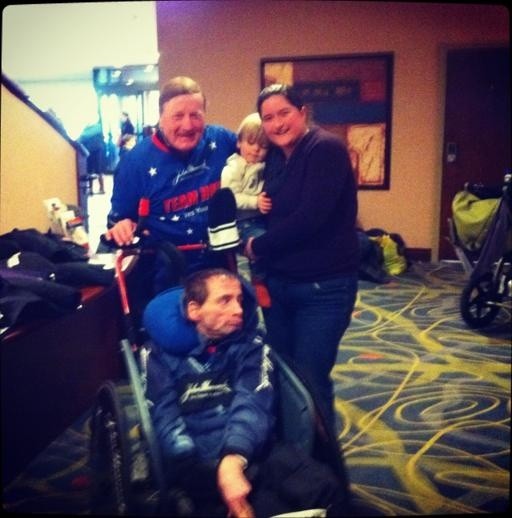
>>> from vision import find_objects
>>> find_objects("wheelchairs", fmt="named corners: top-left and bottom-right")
top-left (94, 230), bottom-right (349, 515)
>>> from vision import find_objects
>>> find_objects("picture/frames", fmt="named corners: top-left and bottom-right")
top-left (259, 51), bottom-right (394, 191)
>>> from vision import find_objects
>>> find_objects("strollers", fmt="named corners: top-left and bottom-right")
top-left (443, 172), bottom-right (512, 329)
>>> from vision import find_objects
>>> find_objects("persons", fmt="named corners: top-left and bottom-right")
top-left (219, 114), bottom-right (273, 309)
top-left (120, 112), bottom-right (135, 136)
top-left (147, 266), bottom-right (350, 518)
top-left (105, 76), bottom-right (240, 279)
top-left (241, 84), bottom-right (359, 434)
